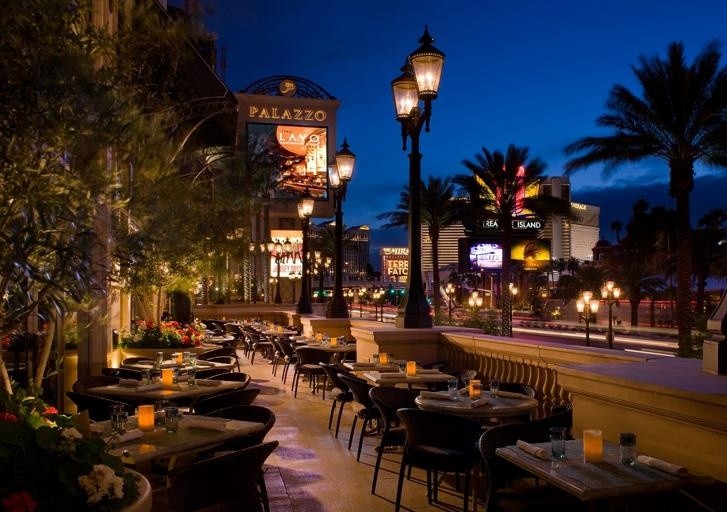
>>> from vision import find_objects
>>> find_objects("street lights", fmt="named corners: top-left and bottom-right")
top-left (445, 282), bottom-right (456, 325)
top-left (342, 287), bottom-right (387, 323)
top-left (575, 289), bottom-right (600, 348)
top-left (468, 290), bottom-right (483, 308)
top-left (325, 136), bottom-right (357, 318)
top-left (599, 280), bottom-right (622, 350)
top-left (390, 21), bottom-right (447, 329)
top-left (296, 185), bottom-right (316, 315)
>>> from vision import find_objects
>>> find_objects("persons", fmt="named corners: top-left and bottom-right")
top-left (275, 239), bottom-right (283, 264)
top-left (283, 238), bottom-right (293, 264)
top-left (292, 239), bottom-right (302, 265)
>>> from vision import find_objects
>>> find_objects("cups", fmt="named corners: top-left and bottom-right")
top-left (166, 407), bottom-right (178, 434)
top-left (151, 369), bottom-right (161, 383)
top-left (157, 352), bottom-right (164, 364)
top-left (398, 360), bottom-right (407, 375)
top-left (171, 354), bottom-right (178, 367)
top-left (141, 372), bottom-right (150, 385)
top-left (386, 352), bottom-right (394, 364)
top-left (115, 412), bottom-right (128, 434)
top-left (448, 377), bottom-right (457, 400)
top-left (373, 352), bottom-right (380, 367)
top-left (473, 384), bottom-right (483, 400)
top-left (172, 370), bottom-right (179, 383)
top-left (488, 380), bottom-right (499, 400)
top-left (550, 427), bottom-right (566, 458)
top-left (183, 351), bottom-right (190, 363)
top-left (187, 370), bottom-right (196, 384)
top-left (617, 433), bottom-right (637, 464)
top-left (190, 353), bottom-right (196, 367)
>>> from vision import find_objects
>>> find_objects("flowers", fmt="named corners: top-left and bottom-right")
top-left (0, 385), bottom-right (140, 512)
